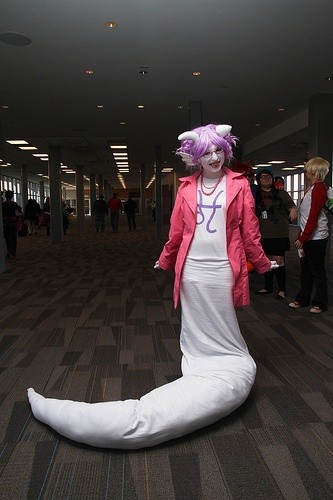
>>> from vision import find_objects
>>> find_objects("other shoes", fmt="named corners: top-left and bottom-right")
top-left (309, 306), bottom-right (322, 313)
top-left (254, 289), bottom-right (273, 295)
top-left (288, 301), bottom-right (301, 307)
top-left (275, 291), bottom-right (286, 300)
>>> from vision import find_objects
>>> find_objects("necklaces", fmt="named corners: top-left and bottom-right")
top-left (201, 172), bottom-right (225, 196)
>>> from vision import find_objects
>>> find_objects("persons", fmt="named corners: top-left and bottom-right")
top-left (152, 201), bottom-right (156, 222)
top-left (232, 156), bottom-right (333, 313)
top-left (124, 194), bottom-right (137, 231)
top-left (92, 195), bottom-right (109, 232)
top-left (26, 123), bottom-right (280, 449)
top-left (0, 190), bottom-right (72, 259)
top-left (107, 193), bottom-right (123, 232)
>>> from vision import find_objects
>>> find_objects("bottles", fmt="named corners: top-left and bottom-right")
top-left (295, 240), bottom-right (306, 263)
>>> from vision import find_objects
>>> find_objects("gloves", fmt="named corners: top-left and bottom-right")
top-left (153, 260), bottom-right (161, 270)
top-left (266, 260), bottom-right (279, 272)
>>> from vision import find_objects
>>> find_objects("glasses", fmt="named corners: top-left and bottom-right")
top-left (261, 175), bottom-right (271, 179)
top-left (197, 148), bottom-right (223, 159)
